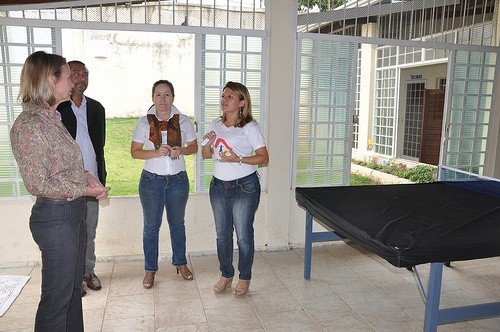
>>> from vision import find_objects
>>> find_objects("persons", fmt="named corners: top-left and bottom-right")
top-left (201, 82), bottom-right (269, 298)
top-left (9, 51), bottom-right (111, 332)
top-left (55, 61), bottom-right (105, 295)
top-left (131, 81), bottom-right (199, 289)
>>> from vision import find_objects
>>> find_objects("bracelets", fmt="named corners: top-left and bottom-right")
top-left (239, 155), bottom-right (242, 165)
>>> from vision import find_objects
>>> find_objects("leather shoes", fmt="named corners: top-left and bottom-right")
top-left (83, 273), bottom-right (101, 290)
top-left (82, 289), bottom-right (86, 297)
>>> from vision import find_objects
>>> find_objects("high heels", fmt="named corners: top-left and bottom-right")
top-left (213, 277), bottom-right (232, 292)
top-left (235, 280), bottom-right (250, 297)
top-left (143, 272), bottom-right (155, 288)
top-left (176, 265), bottom-right (193, 280)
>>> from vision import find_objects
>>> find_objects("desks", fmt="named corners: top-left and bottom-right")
top-left (294, 179), bottom-right (500, 332)
